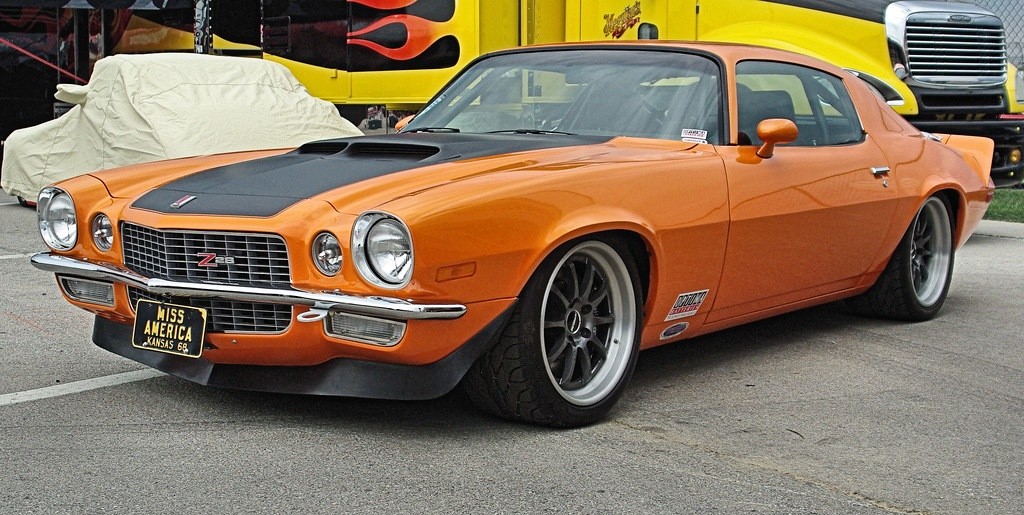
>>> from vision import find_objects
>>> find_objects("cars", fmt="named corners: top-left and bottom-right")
top-left (2, 52), bottom-right (363, 210)
top-left (29, 37), bottom-right (995, 431)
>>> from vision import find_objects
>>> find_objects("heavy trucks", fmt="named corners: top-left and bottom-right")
top-left (0, 0), bottom-right (1022, 194)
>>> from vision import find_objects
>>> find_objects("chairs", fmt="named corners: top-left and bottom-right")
top-left (560, 77), bottom-right (800, 144)
